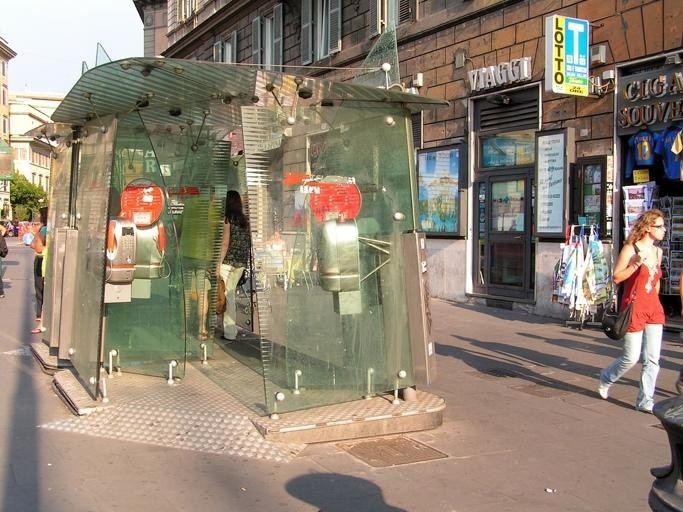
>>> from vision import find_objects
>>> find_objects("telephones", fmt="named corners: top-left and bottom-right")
top-left (106, 220), bottom-right (136, 268)
top-left (158, 220), bottom-right (165, 252)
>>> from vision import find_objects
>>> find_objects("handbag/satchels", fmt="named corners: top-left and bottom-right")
top-left (600, 305), bottom-right (634, 338)
top-left (210, 276), bottom-right (226, 315)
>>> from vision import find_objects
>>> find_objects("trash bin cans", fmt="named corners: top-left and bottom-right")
top-left (18, 221), bottom-right (42, 243)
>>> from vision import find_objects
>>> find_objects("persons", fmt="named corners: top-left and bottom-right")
top-left (261, 230), bottom-right (287, 256)
top-left (175, 185), bottom-right (219, 342)
top-left (29, 232), bottom-right (46, 334)
top-left (0, 224), bottom-right (7, 298)
top-left (598, 209), bottom-right (666, 414)
top-left (674, 268), bottom-right (682, 397)
top-left (214, 190), bottom-right (251, 341)
top-left (26, 207), bottom-right (47, 321)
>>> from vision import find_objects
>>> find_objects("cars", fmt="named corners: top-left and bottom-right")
top-left (0, 219), bottom-right (44, 240)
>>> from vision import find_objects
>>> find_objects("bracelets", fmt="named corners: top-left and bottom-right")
top-left (215, 263), bottom-right (220, 268)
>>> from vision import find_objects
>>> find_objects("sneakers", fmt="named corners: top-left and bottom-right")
top-left (635, 398), bottom-right (655, 414)
top-left (182, 330), bottom-right (235, 341)
top-left (30, 327), bottom-right (45, 335)
top-left (597, 374), bottom-right (610, 399)
